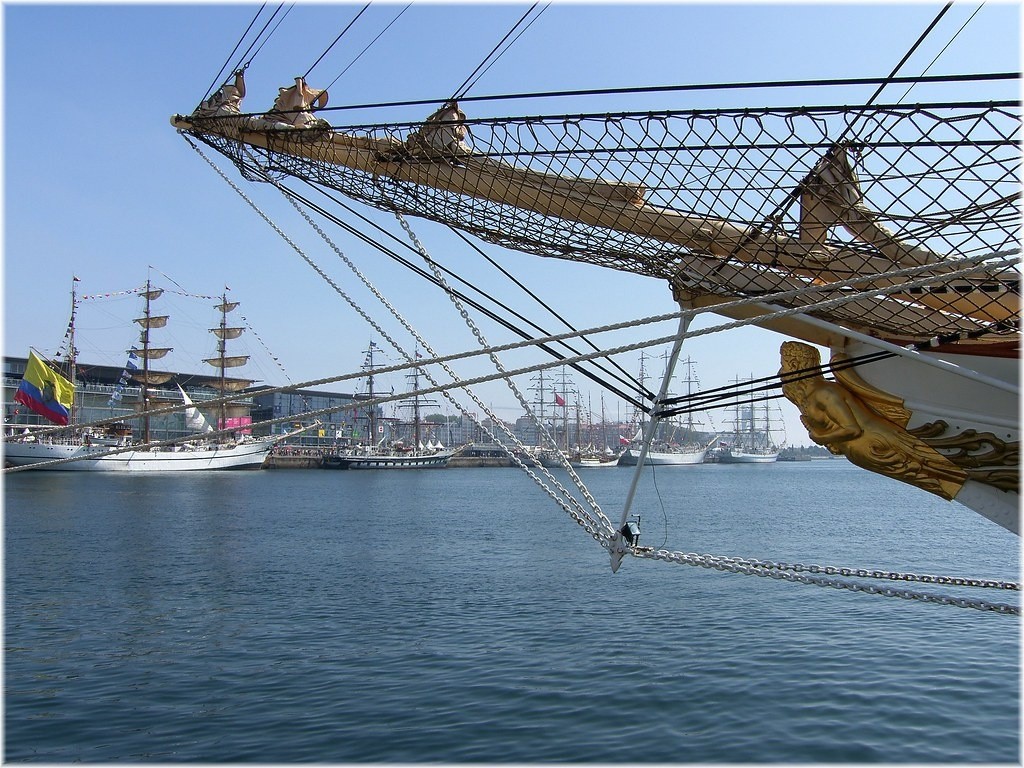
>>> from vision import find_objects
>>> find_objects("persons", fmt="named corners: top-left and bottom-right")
top-left (126, 438), bottom-right (145, 448)
top-left (482, 452), bottom-right (496, 457)
top-left (271, 446), bottom-right (333, 456)
top-left (87, 440), bottom-right (92, 447)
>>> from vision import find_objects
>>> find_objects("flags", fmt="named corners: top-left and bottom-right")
top-left (120, 378), bottom-right (127, 384)
top-left (619, 434), bottom-right (629, 445)
top-left (130, 352), bottom-right (138, 361)
top-left (116, 385), bottom-right (124, 392)
top-left (123, 370), bottom-right (132, 378)
top-left (111, 391), bottom-right (123, 401)
top-left (126, 361), bottom-right (137, 370)
top-left (105, 399), bottom-right (116, 406)
top-left (555, 392), bottom-right (565, 407)
top-left (13, 352), bottom-right (77, 426)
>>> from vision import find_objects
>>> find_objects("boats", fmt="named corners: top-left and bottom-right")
top-left (509, 347), bottom-right (787, 469)
top-left (262, 339), bottom-right (462, 469)
top-left (4, 264), bottom-right (320, 478)
